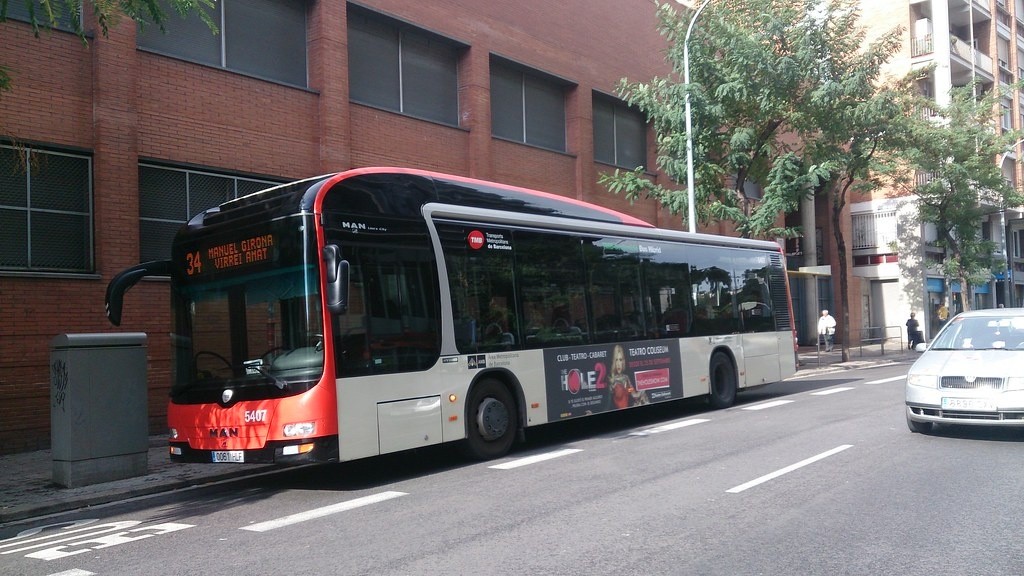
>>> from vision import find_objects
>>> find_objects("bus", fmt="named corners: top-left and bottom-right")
top-left (103, 164), bottom-right (802, 468)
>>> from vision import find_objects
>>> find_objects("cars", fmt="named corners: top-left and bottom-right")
top-left (906, 308), bottom-right (1024, 433)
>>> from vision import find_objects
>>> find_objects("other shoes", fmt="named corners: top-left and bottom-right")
top-left (908, 345), bottom-right (916, 350)
top-left (825, 349), bottom-right (834, 352)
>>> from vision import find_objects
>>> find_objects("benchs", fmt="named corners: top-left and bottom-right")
top-left (530, 317), bottom-right (582, 336)
top-left (479, 323), bottom-right (514, 345)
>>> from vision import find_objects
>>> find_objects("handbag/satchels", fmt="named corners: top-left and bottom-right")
top-left (826, 327), bottom-right (836, 335)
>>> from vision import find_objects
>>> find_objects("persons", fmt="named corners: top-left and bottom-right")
top-left (817, 310), bottom-right (837, 352)
top-left (936, 303), bottom-right (949, 330)
top-left (906, 313), bottom-right (919, 350)
top-left (609, 345), bottom-right (636, 409)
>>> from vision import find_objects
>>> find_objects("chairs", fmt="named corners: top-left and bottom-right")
top-left (366, 299), bottom-right (401, 345)
